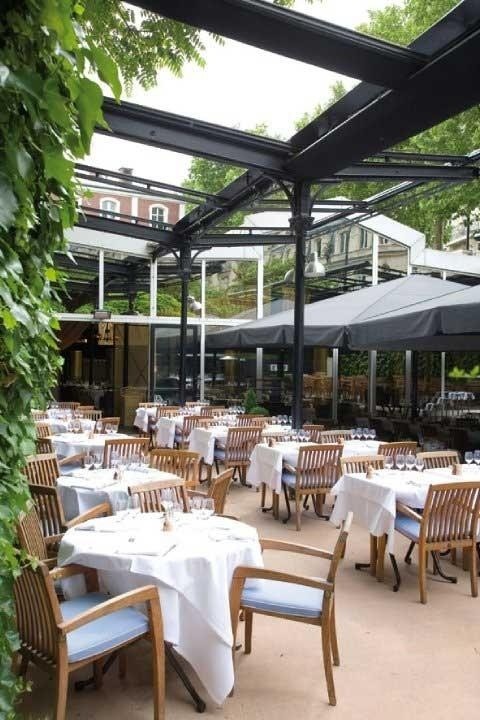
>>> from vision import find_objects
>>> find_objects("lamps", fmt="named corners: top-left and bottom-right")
top-left (187, 296), bottom-right (201, 310)
top-left (284, 264), bottom-right (296, 284)
top-left (303, 252), bottom-right (326, 278)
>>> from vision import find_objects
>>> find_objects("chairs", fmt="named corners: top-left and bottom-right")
top-left (63, 502), bottom-right (111, 531)
top-left (4, 545), bottom-right (167, 720)
top-left (11, 500), bottom-right (65, 570)
top-left (416, 450), bottom-right (460, 470)
top-left (377, 482), bottom-right (480, 603)
top-left (224, 512), bottom-right (354, 705)
top-left (26, 484), bottom-right (63, 539)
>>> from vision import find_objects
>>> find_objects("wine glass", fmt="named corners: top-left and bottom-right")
top-left (369, 429), bottom-right (376, 443)
top-left (416, 455), bottom-right (425, 476)
top-left (465, 451), bottom-right (474, 469)
top-left (357, 427), bottom-right (363, 443)
top-left (363, 427), bottom-right (369, 443)
top-left (407, 455), bottom-right (416, 473)
top-left (396, 455), bottom-right (406, 474)
top-left (474, 450), bottom-right (480, 469)
top-left (45, 398), bottom-right (312, 530)
top-left (384, 456), bottom-right (394, 474)
top-left (350, 428), bottom-right (357, 443)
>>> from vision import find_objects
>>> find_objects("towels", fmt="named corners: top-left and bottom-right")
top-left (115, 541), bottom-right (175, 557)
